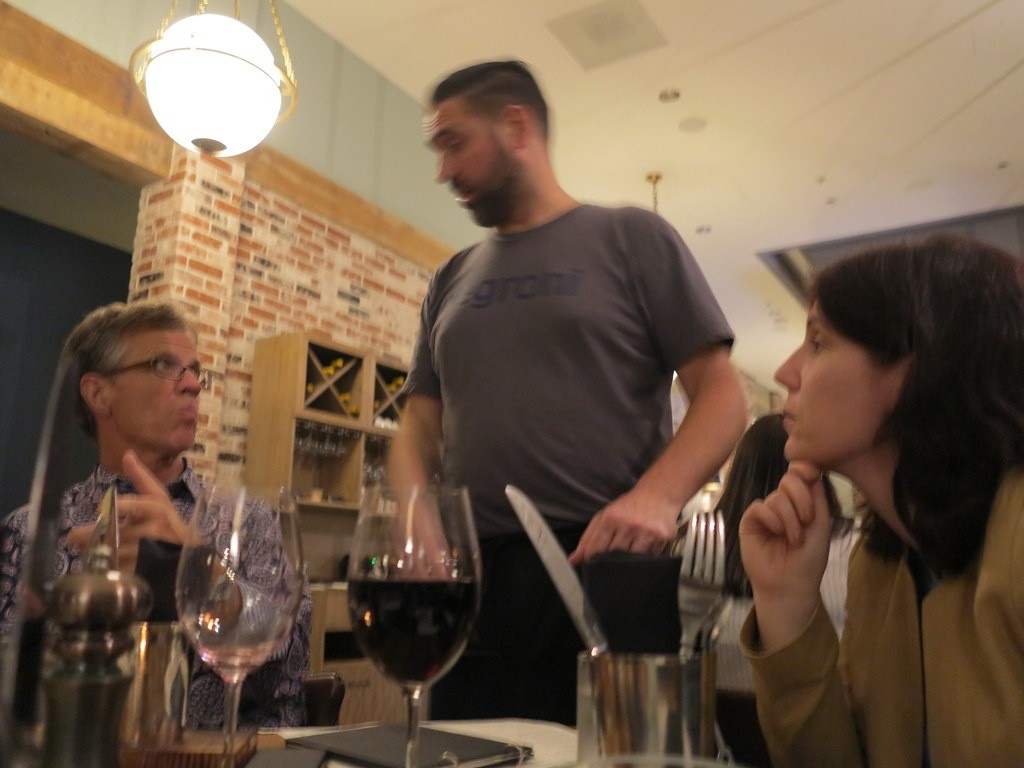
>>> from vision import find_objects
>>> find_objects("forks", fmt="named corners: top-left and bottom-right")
top-left (677, 509), bottom-right (725, 655)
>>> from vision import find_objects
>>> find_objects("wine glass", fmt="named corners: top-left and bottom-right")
top-left (174, 485), bottom-right (304, 768)
top-left (347, 485), bottom-right (482, 768)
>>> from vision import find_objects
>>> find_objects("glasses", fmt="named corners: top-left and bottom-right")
top-left (102, 353), bottom-right (213, 394)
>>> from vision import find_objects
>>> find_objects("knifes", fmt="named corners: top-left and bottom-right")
top-left (504, 484), bottom-right (609, 657)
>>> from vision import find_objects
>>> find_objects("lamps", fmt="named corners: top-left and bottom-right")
top-left (129, 0), bottom-right (299, 157)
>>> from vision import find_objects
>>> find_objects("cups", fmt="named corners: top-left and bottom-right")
top-left (576, 651), bottom-right (717, 768)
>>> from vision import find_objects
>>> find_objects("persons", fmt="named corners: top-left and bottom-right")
top-left (678, 234), bottom-right (1024, 768)
top-left (390, 59), bottom-right (748, 730)
top-left (0, 302), bottom-right (314, 730)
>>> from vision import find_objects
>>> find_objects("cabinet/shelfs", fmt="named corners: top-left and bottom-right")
top-left (243, 333), bottom-right (411, 677)
